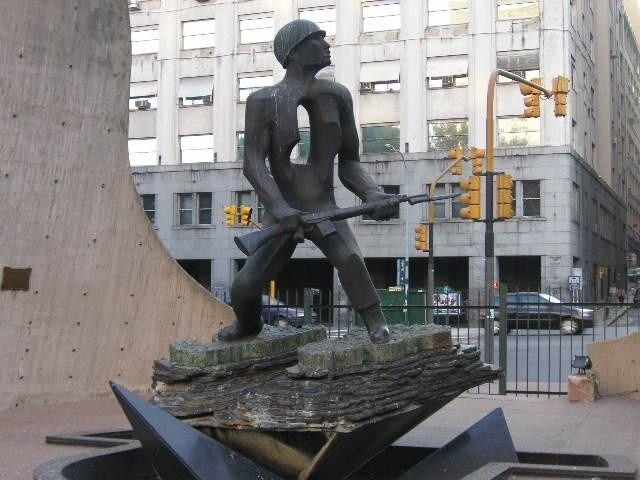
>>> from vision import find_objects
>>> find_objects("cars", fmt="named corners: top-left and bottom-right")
top-left (480, 290), bottom-right (595, 336)
top-left (225, 292), bottom-right (319, 332)
top-left (627, 267), bottom-right (640, 306)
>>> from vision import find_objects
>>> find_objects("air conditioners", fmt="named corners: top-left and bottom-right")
top-left (135, 99), bottom-right (149, 109)
top-left (361, 82), bottom-right (372, 90)
top-left (513, 70), bottom-right (526, 82)
top-left (442, 74), bottom-right (455, 87)
top-left (203, 94), bottom-right (214, 104)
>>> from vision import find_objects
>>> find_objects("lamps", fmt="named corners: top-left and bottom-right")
top-left (571, 353), bottom-right (593, 375)
top-left (384, 142), bottom-right (409, 169)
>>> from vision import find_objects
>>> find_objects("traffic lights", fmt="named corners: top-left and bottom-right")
top-left (413, 222), bottom-right (427, 250)
top-left (223, 205), bottom-right (236, 226)
top-left (240, 204), bottom-right (253, 226)
top-left (555, 75), bottom-right (571, 117)
top-left (496, 173), bottom-right (514, 219)
top-left (471, 145), bottom-right (486, 175)
top-left (520, 77), bottom-right (541, 118)
top-left (459, 173), bottom-right (480, 219)
top-left (447, 146), bottom-right (462, 177)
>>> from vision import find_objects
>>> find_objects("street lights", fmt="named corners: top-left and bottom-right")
top-left (382, 143), bottom-right (413, 323)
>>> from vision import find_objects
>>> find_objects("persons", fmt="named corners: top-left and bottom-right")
top-left (609, 284), bottom-right (618, 302)
top-left (618, 283), bottom-right (625, 302)
top-left (218, 21), bottom-right (400, 343)
